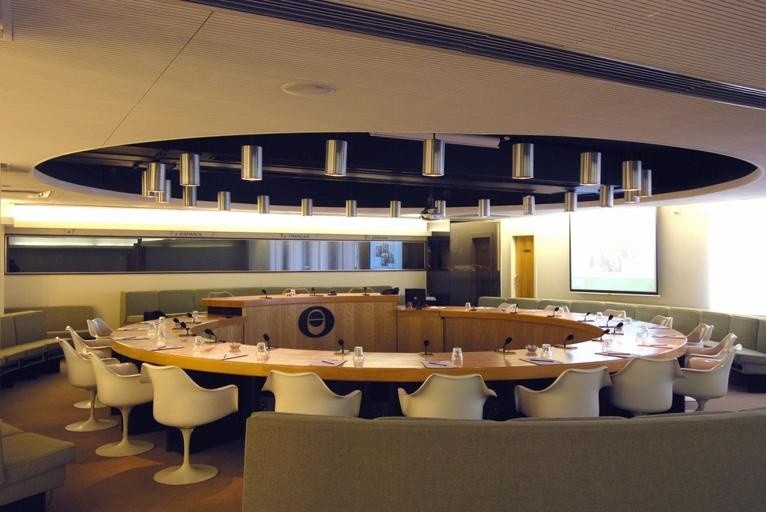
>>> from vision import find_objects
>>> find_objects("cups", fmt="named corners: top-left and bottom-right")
top-left (191, 336), bottom-right (204, 356)
top-left (255, 342), bottom-right (266, 355)
top-left (352, 345), bottom-right (363, 359)
top-left (407, 302), bottom-right (412, 309)
top-left (542, 343), bottom-right (552, 357)
top-left (595, 311), bottom-right (649, 352)
top-left (148, 316), bottom-right (167, 344)
top-left (557, 307), bottom-right (564, 316)
top-left (451, 346), bottom-right (464, 361)
top-left (510, 305), bottom-right (515, 312)
top-left (465, 302), bottom-right (471, 311)
top-left (191, 310), bottom-right (199, 318)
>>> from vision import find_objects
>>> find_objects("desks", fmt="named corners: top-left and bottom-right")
top-left (111, 302), bottom-right (688, 382)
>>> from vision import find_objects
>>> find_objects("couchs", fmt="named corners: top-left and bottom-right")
top-left (478, 296), bottom-right (766, 376)
top-left (240, 409), bottom-right (766, 512)
top-left (0, 415), bottom-right (77, 512)
top-left (4, 306), bottom-right (96, 340)
top-left (0, 309), bottom-right (73, 389)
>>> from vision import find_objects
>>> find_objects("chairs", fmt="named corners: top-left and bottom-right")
top-left (87, 352), bottom-right (154, 458)
top-left (515, 365), bottom-right (612, 416)
top-left (398, 372), bottom-right (497, 421)
top-left (142, 361), bottom-right (238, 486)
top-left (610, 357), bottom-right (683, 413)
top-left (65, 317), bottom-right (116, 360)
top-left (649, 315), bottom-right (738, 366)
top-left (261, 369), bottom-right (361, 417)
top-left (56, 335), bottom-right (139, 432)
top-left (673, 342), bottom-right (742, 411)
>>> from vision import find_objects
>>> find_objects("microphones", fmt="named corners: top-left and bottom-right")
top-left (516, 304), bottom-right (592, 321)
top-left (613, 322), bottom-right (623, 333)
top-left (424, 339), bottom-right (429, 355)
top-left (172, 313), bottom-right (192, 335)
top-left (503, 337), bottom-right (511, 350)
top-left (564, 335), bottom-right (573, 346)
top-left (607, 314), bottom-right (613, 326)
top-left (311, 288), bottom-right (319, 295)
top-left (261, 288), bottom-right (269, 297)
top-left (204, 329), bottom-right (218, 342)
top-left (339, 339), bottom-right (345, 353)
top-left (262, 333), bottom-right (271, 349)
top-left (598, 329), bottom-right (609, 337)
top-left (363, 286), bottom-right (368, 295)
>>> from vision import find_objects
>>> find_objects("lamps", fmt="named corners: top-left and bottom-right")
top-left (217, 191), bottom-right (231, 212)
top-left (479, 199), bottom-right (491, 217)
top-left (240, 145), bottom-right (263, 182)
top-left (390, 200), bottom-right (401, 219)
top-left (564, 192), bottom-right (577, 213)
top-left (622, 160), bottom-right (653, 205)
top-left (345, 200), bottom-right (357, 218)
top-left (434, 200), bottom-right (446, 218)
top-left (301, 198), bottom-right (313, 217)
top-left (422, 138), bottom-right (445, 177)
top-left (324, 140), bottom-right (348, 177)
top-left (579, 151), bottom-right (602, 186)
top-left (600, 185), bottom-right (614, 208)
top-left (257, 195), bottom-right (270, 214)
top-left (511, 142), bottom-right (534, 180)
top-left (182, 185), bottom-right (197, 207)
top-left (178, 153), bottom-right (200, 187)
top-left (141, 161), bottom-right (171, 204)
top-left (523, 195), bottom-right (535, 216)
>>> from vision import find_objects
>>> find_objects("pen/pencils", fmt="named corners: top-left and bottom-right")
top-left (159, 345), bottom-right (167, 349)
top-left (322, 360), bottom-right (334, 365)
top-left (124, 336), bottom-right (137, 340)
top-left (430, 362), bottom-right (448, 367)
top-left (530, 359), bottom-right (554, 363)
top-left (650, 344), bottom-right (669, 346)
top-left (224, 354), bottom-right (227, 359)
top-left (608, 352), bottom-right (632, 356)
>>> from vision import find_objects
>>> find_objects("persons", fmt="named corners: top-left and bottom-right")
top-left (382, 243), bottom-right (394, 266)
top-left (376, 246), bottom-right (392, 267)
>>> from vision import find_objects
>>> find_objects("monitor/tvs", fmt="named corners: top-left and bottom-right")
top-left (405, 288), bottom-right (426, 307)
